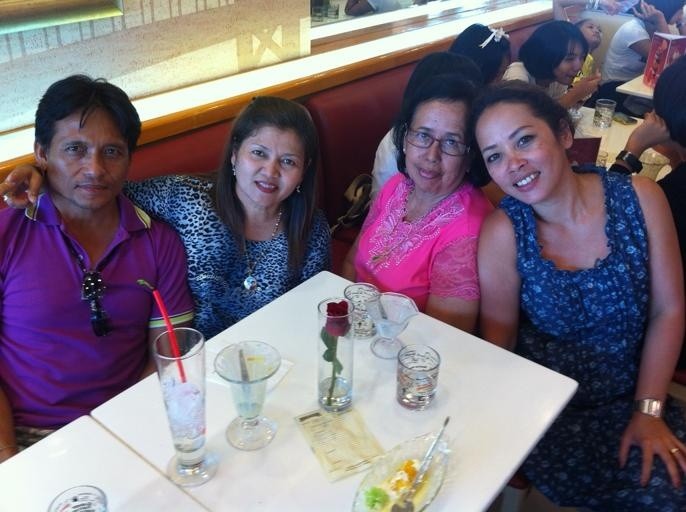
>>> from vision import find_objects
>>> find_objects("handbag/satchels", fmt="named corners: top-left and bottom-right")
top-left (328, 173), bottom-right (372, 239)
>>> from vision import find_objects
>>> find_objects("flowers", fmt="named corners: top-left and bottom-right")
top-left (317, 297), bottom-right (355, 410)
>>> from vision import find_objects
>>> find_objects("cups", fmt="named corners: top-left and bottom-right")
top-left (397, 344), bottom-right (440, 411)
top-left (593, 99), bottom-right (617, 128)
top-left (596, 150), bottom-right (608, 166)
top-left (47, 485), bottom-right (107, 511)
top-left (638, 152), bottom-right (670, 181)
top-left (344, 283), bottom-right (380, 339)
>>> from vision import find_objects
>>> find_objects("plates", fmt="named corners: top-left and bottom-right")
top-left (351, 430), bottom-right (450, 512)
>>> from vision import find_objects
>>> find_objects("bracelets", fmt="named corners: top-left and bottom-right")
top-left (0, 443), bottom-right (18, 455)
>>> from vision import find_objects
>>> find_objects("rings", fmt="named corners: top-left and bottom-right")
top-left (2, 180), bottom-right (14, 188)
top-left (2, 192), bottom-right (14, 202)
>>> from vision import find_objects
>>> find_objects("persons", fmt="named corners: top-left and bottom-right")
top-left (0, 95), bottom-right (334, 355)
top-left (337, 3), bottom-right (686, 512)
top-left (249, 280), bottom-right (255, 290)
top-left (0, 74), bottom-right (196, 465)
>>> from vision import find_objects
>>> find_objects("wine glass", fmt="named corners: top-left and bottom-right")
top-left (150, 326), bottom-right (219, 486)
top-left (214, 340), bottom-right (280, 452)
top-left (367, 291), bottom-right (420, 360)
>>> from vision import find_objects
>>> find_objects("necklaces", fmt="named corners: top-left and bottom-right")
top-left (239, 207), bottom-right (285, 292)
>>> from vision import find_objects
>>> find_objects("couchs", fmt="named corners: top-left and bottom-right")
top-left (0, 9), bottom-right (555, 268)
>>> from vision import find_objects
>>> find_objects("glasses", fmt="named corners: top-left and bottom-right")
top-left (404, 122), bottom-right (473, 156)
